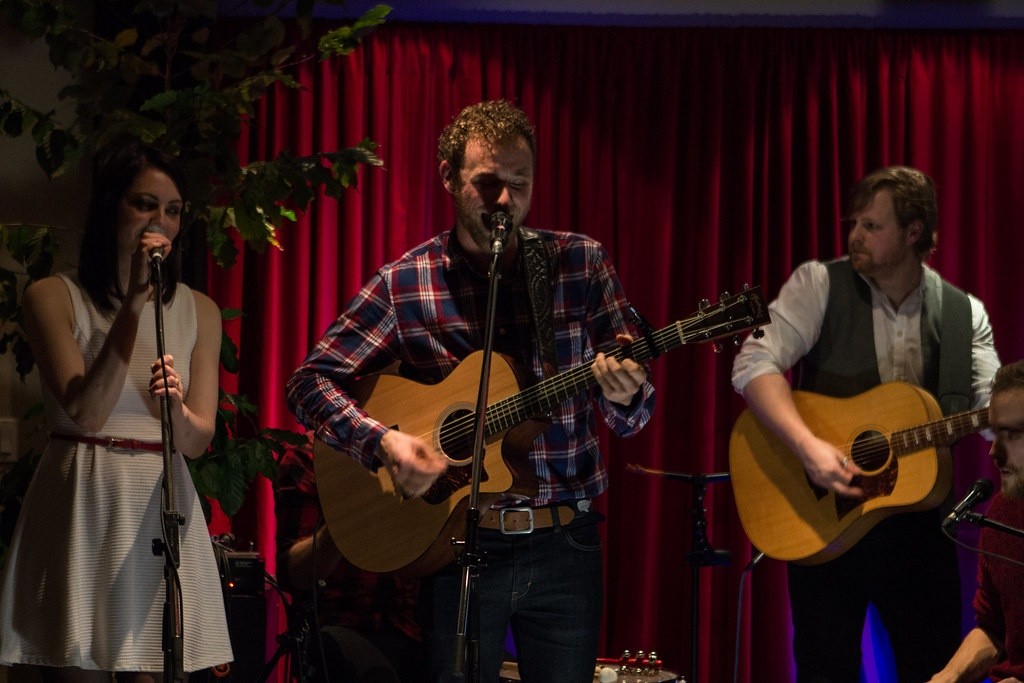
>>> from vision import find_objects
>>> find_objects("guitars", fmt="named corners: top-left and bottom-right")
top-left (729, 379), bottom-right (990, 568)
top-left (314, 284), bottom-right (772, 581)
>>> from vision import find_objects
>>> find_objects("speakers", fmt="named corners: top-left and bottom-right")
top-left (187, 595), bottom-right (268, 683)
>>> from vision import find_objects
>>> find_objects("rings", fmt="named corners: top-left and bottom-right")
top-left (841, 457), bottom-right (849, 470)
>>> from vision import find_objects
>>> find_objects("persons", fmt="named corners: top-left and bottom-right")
top-left (285, 99), bottom-right (655, 683)
top-left (731, 165), bottom-right (1003, 683)
top-left (0, 134), bottom-right (235, 683)
top-left (926, 358), bottom-right (1024, 683)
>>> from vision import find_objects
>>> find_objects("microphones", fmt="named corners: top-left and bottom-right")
top-left (941, 477), bottom-right (995, 530)
top-left (487, 211), bottom-right (513, 254)
top-left (143, 225), bottom-right (165, 263)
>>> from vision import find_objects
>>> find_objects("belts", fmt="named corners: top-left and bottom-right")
top-left (50, 428), bottom-right (165, 452)
top-left (479, 497), bottom-right (594, 534)
